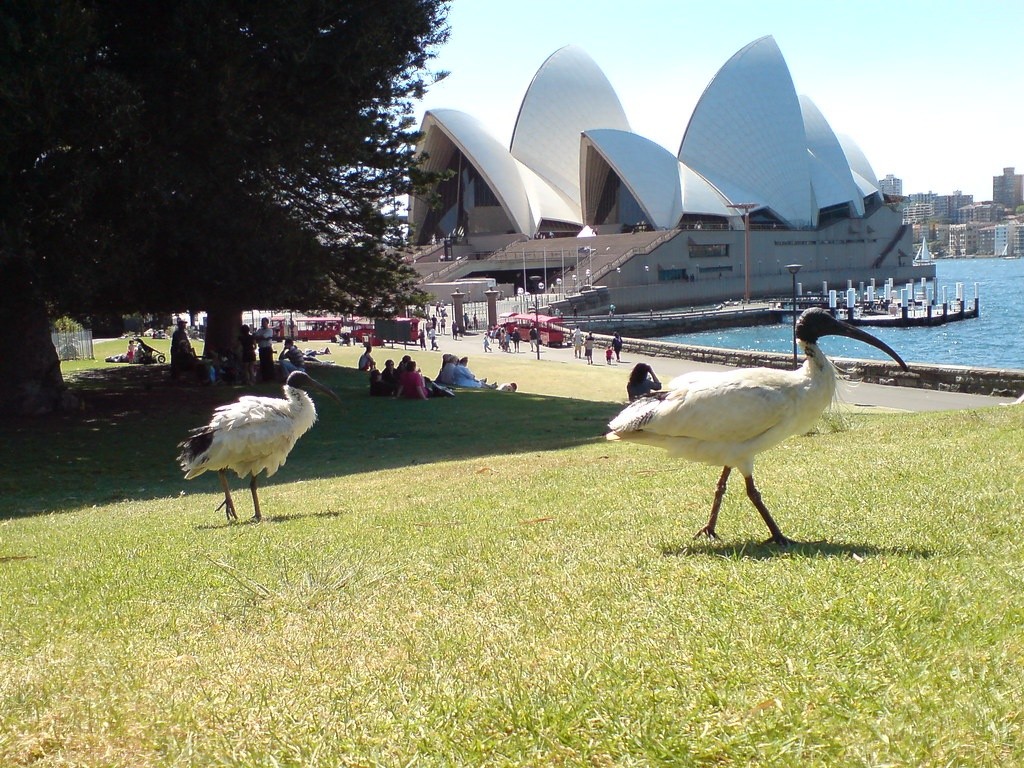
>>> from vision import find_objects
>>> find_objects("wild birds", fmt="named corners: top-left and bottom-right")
top-left (174, 371), bottom-right (342, 523)
top-left (604, 307), bottom-right (910, 547)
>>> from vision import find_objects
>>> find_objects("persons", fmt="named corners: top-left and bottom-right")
top-left (551, 283), bottom-right (555, 293)
top-left (627, 364), bottom-right (661, 403)
top-left (534, 218), bottom-right (777, 240)
top-left (126, 299), bottom-right (625, 402)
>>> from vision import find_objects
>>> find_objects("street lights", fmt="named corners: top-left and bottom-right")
top-left (783, 263), bottom-right (803, 370)
top-left (517, 287), bottom-right (524, 314)
top-left (538, 282), bottom-right (544, 306)
top-left (572, 274), bottom-right (577, 294)
top-left (586, 272), bottom-right (591, 287)
top-left (555, 279), bottom-right (562, 301)
top-left (725, 202), bottom-right (762, 301)
top-left (528, 273), bottom-right (541, 360)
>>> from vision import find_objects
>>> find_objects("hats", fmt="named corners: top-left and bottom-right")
top-left (177, 320), bottom-right (188, 325)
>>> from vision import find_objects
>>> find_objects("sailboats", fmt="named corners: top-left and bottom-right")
top-left (911, 236), bottom-right (936, 263)
top-left (1001, 244), bottom-right (1019, 260)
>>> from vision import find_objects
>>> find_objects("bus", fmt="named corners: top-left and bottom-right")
top-left (269, 317), bottom-right (344, 342)
top-left (514, 315), bottom-right (565, 348)
top-left (350, 317), bottom-right (420, 344)
